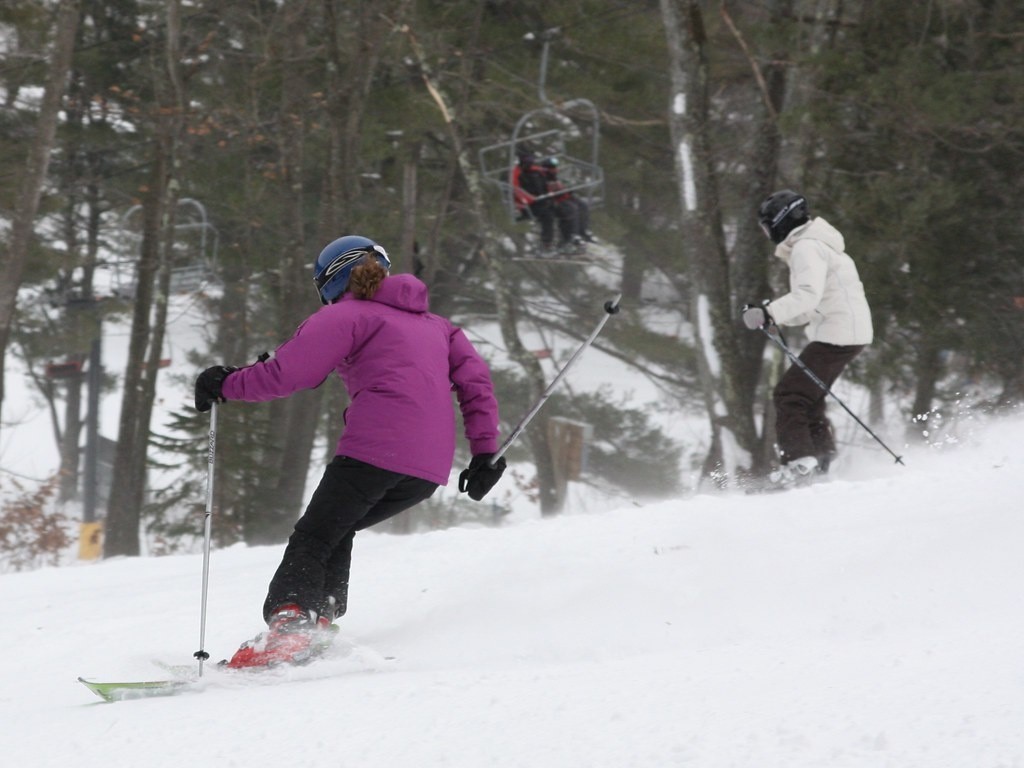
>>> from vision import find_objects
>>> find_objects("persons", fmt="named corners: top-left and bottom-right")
top-left (512, 143), bottom-right (579, 254)
top-left (541, 158), bottom-right (597, 245)
top-left (742, 189), bottom-right (874, 488)
top-left (194, 235), bottom-right (507, 669)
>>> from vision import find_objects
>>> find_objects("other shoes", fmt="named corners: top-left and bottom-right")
top-left (583, 230), bottom-right (599, 243)
top-left (559, 241), bottom-right (578, 256)
top-left (571, 234), bottom-right (587, 248)
top-left (536, 244), bottom-right (558, 258)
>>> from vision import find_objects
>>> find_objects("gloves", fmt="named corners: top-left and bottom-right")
top-left (194, 364), bottom-right (240, 412)
top-left (467, 453), bottom-right (507, 502)
top-left (743, 308), bottom-right (775, 331)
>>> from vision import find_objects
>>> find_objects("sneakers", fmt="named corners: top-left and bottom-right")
top-left (765, 456), bottom-right (819, 491)
top-left (228, 603), bottom-right (340, 670)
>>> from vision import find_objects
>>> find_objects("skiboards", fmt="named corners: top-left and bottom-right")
top-left (513, 252), bottom-right (601, 264)
top-left (74, 657), bottom-right (389, 702)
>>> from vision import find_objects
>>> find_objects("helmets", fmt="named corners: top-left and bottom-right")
top-left (543, 157), bottom-right (560, 168)
top-left (757, 190), bottom-right (809, 244)
top-left (312, 235), bottom-right (392, 306)
